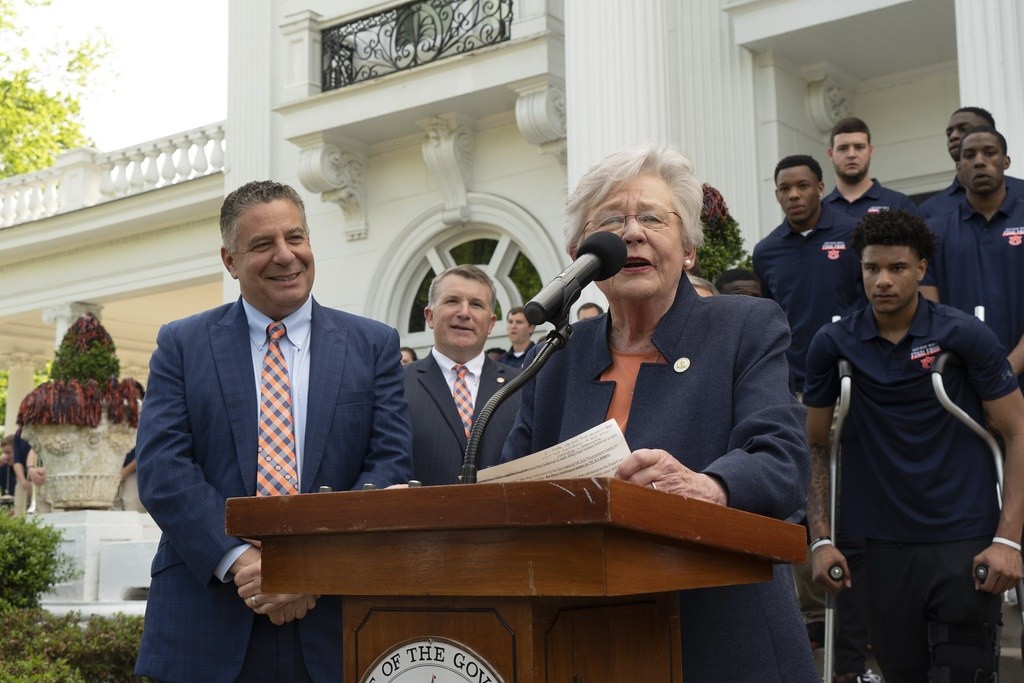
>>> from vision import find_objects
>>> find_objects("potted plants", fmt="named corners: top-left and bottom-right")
top-left (16, 313), bottom-right (145, 508)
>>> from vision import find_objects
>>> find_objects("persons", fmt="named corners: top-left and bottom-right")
top-left (577, 303), bottom-right (603, 321)
top-left (918, 106), bottom-right (1024, 211)
top-left (752, 155), bottom-right (866, 392)
top-left (498, 307), bottom-right (536, 368)
top-left (917, 126), bottom-right (1024, 392)
top-left (0, 428), bottom-right (45, 496)
top-left (500, 146), bottom-right (821, 683)
top-left (801, 209), bottom-right (1024, 683)
top-left (400, 347), bottom-right (417, 365)
top-left (822, 117), bottom-right (917, 221)
top-left (404, 264), bottom-right (524, 486)
top-left (688, 269), bottom-right (761, 297)
top-left (135, 178), bottom-right (414, 683)
top-left (120, 381), bottom-right (147, 512)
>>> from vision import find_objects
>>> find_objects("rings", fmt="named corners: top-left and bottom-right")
top-left (652, 481), bottom-right (657, 489)
top-left (252, 596), bottom-right (258, 606)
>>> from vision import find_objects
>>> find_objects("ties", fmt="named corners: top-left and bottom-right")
top-left (453, 365), bottom-right (473, 441)
top-left (256, 322), bottom-right (298, 496)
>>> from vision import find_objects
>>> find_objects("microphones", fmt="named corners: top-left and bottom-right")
top-left (523, 230), bottom-right (628, 326)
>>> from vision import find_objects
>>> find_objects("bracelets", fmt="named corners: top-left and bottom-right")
top-left (810, 536), bottom-right (831, 552)
top-left (992, 537), bottom-right (1021, 551)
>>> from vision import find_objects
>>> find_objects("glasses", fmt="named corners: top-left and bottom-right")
top-left (583, 212), bottom-right (682, 234)
